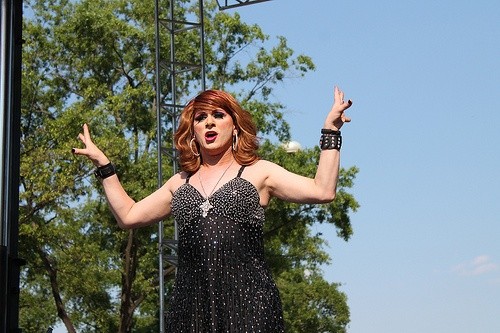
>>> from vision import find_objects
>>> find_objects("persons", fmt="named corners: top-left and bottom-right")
top-left (70, 84), bottom-right (353, 333)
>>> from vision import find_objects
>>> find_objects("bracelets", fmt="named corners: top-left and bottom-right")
top-left (321, 128), bottom-right (340, 135)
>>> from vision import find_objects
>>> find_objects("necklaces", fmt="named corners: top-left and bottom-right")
top-left (198, 159), bottom-right (234, 218)
